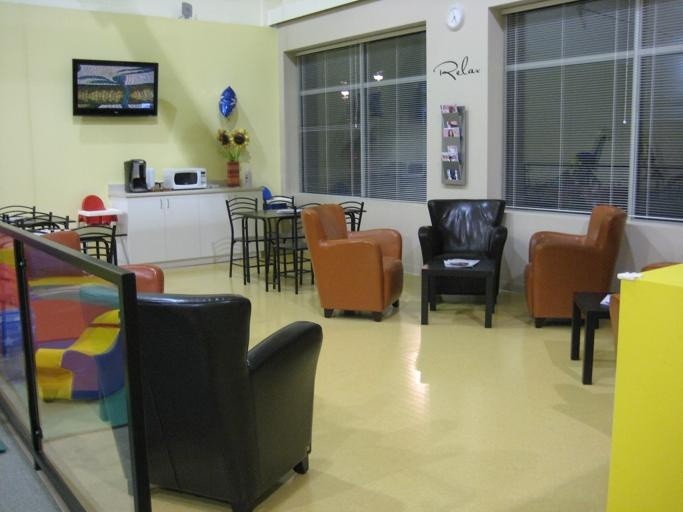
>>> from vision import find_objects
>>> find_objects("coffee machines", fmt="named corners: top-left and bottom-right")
top-left (124, 159), bottom-right (149, 193)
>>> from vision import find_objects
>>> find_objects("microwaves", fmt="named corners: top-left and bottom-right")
top-left (162, 168), bottom-right (207, 190)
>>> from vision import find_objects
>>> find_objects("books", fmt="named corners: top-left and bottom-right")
top-left (439, 103), bottom-right (464, 182)
top-left (443, 257), bottom-right (480, 269)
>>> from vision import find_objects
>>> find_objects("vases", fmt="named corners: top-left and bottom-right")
top-left (224, 158), bottom-right (241, 188)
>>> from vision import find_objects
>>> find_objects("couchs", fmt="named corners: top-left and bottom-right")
top-left (0, 228), bottom-right (167, 403)
top-left (77, 284), bottom-right (325, 509)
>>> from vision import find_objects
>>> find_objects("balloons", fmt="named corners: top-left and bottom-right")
top-left (218, 86), bottom-right (236, 119)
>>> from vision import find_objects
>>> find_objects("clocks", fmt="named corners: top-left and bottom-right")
top-left (445, 5), bottom-right (465, 31)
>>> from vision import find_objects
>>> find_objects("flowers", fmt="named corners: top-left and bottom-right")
top-left (214, 125), bottom-right (250, 165)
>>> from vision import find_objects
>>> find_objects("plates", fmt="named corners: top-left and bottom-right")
top-left (276, 209), bottom-right (301, 214)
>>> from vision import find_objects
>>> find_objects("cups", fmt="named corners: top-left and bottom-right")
top-left (152, 181), bottom-right (164, 192)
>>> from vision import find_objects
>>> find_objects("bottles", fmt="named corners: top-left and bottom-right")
top-left (245, 167), bottom-right (252, 189)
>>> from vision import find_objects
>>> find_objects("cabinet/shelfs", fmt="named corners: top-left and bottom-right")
top-left (435, 104), bottom-right (464, 187)
top-left (605, 262), bottom-right (683, 512)
top-left (106, 185), bottom-right (265, 275)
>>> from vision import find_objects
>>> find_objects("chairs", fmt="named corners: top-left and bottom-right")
top-left (299, 202), bottom-right (402, 323)
top-left (414, 196), bottom-right (508, 330)
top-left (0, 205), bottom-right (129, 268)
top-left (223, 192), bottom-right (366, 301)
top-left (521, 202), bottom-right (627, 332)
top-left (605, 261), bottom-right (679, 347)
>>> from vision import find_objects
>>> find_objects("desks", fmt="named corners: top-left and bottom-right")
top-left (568, 288), bottom-right (618, 387)
top-left (419, 258), bottom-right (500, 328)
